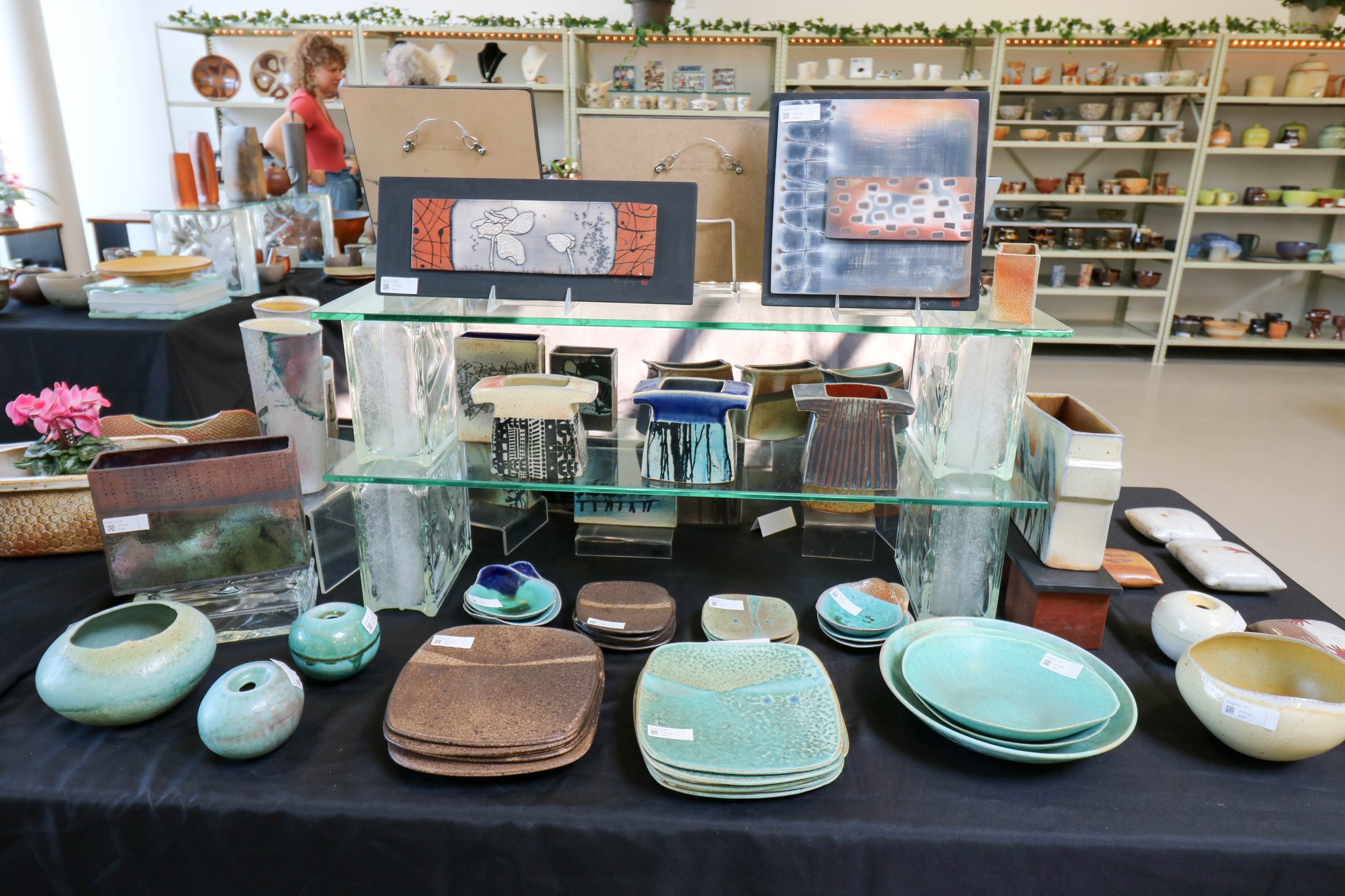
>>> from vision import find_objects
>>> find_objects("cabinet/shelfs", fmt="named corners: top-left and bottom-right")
top-left (156, 20), bottom-right (362, 210)
top-left (568, 26), bottom-right (783, 178)
top-left (1121, 30), bottom-right (1345, 367)
top-left (356, 24), bottom-right (572, 175)
top-left (987, 32), bottom-right (1224, 363)
top-left (781, 30), bottom-right (1000, 88)
top-left (309, 279), bottom-right (1076, 623)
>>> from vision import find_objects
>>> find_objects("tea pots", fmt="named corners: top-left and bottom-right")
top-left (264, 161), bottom-right (300, 196)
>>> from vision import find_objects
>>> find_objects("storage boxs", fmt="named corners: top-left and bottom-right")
top-left (85, 434), bottom-right (312, 601)
top-left (988, 242), bottom-right (1041, 324)
top-left (1011, 388), bottom-right (1130, 573)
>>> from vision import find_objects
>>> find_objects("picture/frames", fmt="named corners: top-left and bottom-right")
top-left (375, 176), bottom-right (698, 308)
top-left (574, 111), bottom-right (771, 285)
top-left (759, 92), bottom-right (991, 313)
top-left (336, 82), bottom-right (544, 241)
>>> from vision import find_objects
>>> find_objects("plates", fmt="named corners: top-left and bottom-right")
top-left (879, 616), bottom-right (1138, 764)
top-left (250, 49), bottom-right (295, 99)
top-left (815, 577), bottom-right (915, 647)
top-left (700, 593), bottom-right (800, 644)
top-left (462, 560), bottom-right (562, 627)
top-left (191, 55), bottom-right (240, 99)
top-left (95, 256), bottom-right (213, 282)
top-left (633, 642), bottom-right (850, 798)
top-left (571, 581), bottom-right (677, 651)
top-left (383, 625), bottom-right (605, 777)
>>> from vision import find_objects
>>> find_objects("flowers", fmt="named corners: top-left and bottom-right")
top-left (0, 172), bottom-right (60, 219)
top-left (5, 378), bottom-right (126, 479)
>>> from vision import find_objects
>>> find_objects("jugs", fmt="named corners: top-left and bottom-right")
top-left (577, 78), bottom-right (614, 108)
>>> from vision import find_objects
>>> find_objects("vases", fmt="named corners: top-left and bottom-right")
top-left (0, 408), bottom-right (262, 558)
top-left (0, 212), bottom-right (21, 230)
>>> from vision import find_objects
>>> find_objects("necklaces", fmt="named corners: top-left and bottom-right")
top-left (433, 47), bottom-right (452, 69)
top-left (480, 50), bottom-right (500, 79)
top-left (523, 49), bottom-right (546, 76)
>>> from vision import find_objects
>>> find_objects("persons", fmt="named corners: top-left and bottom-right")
top-left (262, 34), bottom-right (358, 257)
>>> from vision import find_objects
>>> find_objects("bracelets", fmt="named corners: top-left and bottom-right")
top-left (308, 169), bottom-right (312, 178)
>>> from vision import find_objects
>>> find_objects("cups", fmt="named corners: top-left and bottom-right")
top-left (610, 95), bottom-right (752, 111)
top-left (798, 58), bottom-right (941, 82)
top-left (1171, 309), bottom-right (1345, 342)
top-left (238, 295), bottom-right (340, 495)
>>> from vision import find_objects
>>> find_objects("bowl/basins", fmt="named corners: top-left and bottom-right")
top-left (322, 211), bottom-right (377, 268)
top-left (35, 599), bottom-right (217, 725)
top-left (1175, 631), bottom-right (1345, 762)
top-left (197, 661), bottom-right (304, 756)
top-left (980, 52), bottom-right (1345, 289)
top-left (256, 245), bottom-right (300, 285)
top-left (0, 247), bottom-right (154, 310)
top-left (289, 602), bottom-right (381, 679)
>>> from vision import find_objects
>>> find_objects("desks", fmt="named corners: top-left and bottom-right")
top-left (0, 212), bottom-right (1345, 883)
